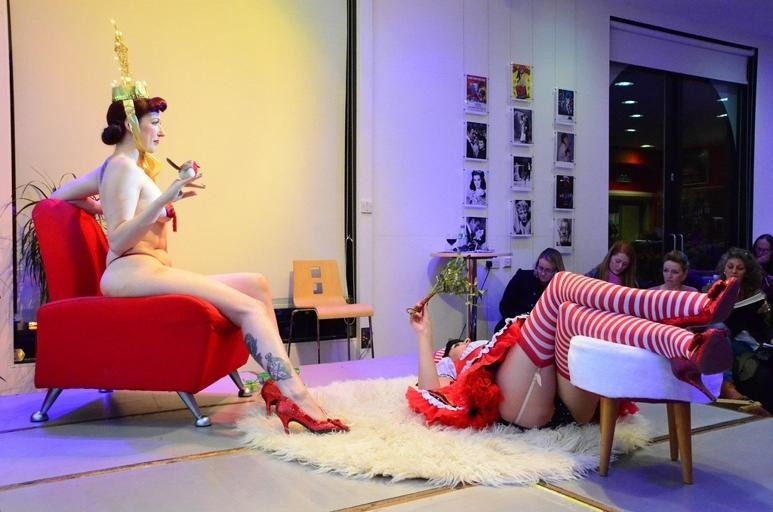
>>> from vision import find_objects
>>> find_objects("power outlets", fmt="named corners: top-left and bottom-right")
top-left (486, 258), bottom-right (498, 268)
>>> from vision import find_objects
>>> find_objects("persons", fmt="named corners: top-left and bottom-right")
top-left (513, 200), bottom-right (531, 235)
top-left (646, 251), bottom-right (698, 292)
top-left (520, 116), bottom-right (530, 143)
top-left (701, 245), bottom-right (773, 413)
top-left (405, 270), bottom-right (739, 432)
top-left (473, 228), bottom-right (485, 248)
top-left (468, 171), bottom-right (486, 205)
top-left (555, 219), bottom-right (571, 247)
top-left (753, 234), bottom-right (773, 303)
top-left (49, 97), bottom-right (349, 435)
top-left (467, 126), bottom-right (486, 159)
top-left (559, 134), bottom-right (570, 162)
top-left (494, 248), bottom-right (565, 335)
top-left (466, 218), bottom-right (481, 243)
top-left (583, 240), bottom-right (637, 287)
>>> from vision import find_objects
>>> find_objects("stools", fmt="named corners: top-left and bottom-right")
top-left (568, 335), bottom-right (725, 484)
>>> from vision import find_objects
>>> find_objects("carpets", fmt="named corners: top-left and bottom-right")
top-left (234, 375), bottom-right (652, 489)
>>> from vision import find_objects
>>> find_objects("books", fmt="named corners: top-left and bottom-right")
top-left (733, 292), bottom-right (765, 323)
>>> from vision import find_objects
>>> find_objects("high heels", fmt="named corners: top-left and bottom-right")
top-left (261, 378), bottom-right (348, 434)
top-left (663, 276), bottom-right (739, 330)
top-left (670, 328), bottom-right (733, 402)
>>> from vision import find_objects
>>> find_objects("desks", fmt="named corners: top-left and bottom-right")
top-left (433, 251), bottom-right (497, 342)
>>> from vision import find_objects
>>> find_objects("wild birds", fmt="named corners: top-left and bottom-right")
top-left (166, 158), bottom-right (200, 181)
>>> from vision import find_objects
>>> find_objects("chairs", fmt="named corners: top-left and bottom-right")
top-left (286, 259), bottom-right (374, 364)
top-left (29, 199), bottom-right (255, 426)
